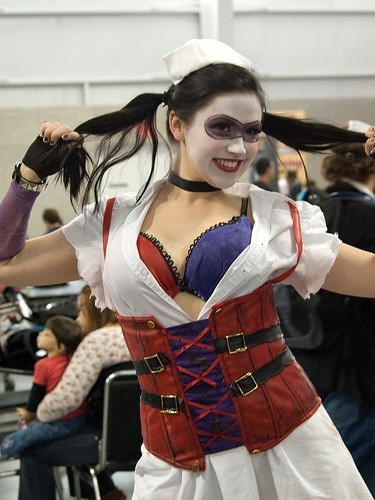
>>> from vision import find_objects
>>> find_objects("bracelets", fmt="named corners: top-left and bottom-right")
top-left (13, 162), bottom-right (46, 193)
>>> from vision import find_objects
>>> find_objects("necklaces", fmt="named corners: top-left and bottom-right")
top-left (168, 170), bottom-right (221, 192)
top-left (326, 190), bottom-right (374, 204)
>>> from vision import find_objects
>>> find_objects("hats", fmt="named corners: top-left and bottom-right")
top-left (348, 120), bottom-right (373, 134)
top-left (162, 38), bottom-right (257, 87)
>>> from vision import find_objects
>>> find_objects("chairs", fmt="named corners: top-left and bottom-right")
top-left (52, 370), bottom-right (144, 500)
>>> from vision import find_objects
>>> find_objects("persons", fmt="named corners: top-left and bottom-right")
top-left (43, 209), bottom-right (65, 234)
top-left (252, 157), bottom-right (276, 191)
top-left (18, 285), bottom-right (133, 499)
top-left (0, 315), bottom-right (93, 460)
top-left (1, 39), bottom-right (375, 500)
top-left (292, 120), bottom-right (375, 500)
top-left (278, 170), bottom-right (327, 207)
